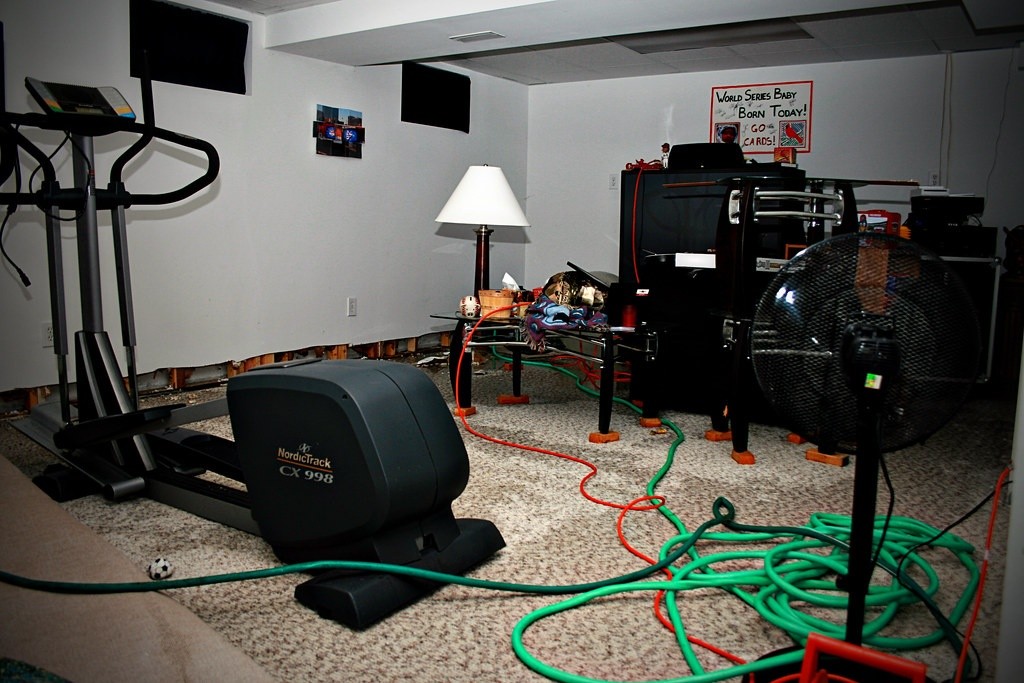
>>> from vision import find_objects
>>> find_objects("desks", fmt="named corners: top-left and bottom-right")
top-left (430, 310), bottom-right (668, 443)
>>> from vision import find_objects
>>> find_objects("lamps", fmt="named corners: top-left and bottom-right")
top-left (435, 164), bottom-right (531, 356)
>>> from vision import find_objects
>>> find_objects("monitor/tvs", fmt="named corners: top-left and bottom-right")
top-left (42, 80), bottom-right (119, 116)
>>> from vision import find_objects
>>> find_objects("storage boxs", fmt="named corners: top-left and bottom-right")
top-left (751, 256), bottom-right (1002, 384)
top-left (857, 210), bottom-right (901, 243)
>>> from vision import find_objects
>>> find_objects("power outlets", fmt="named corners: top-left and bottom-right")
top-left (42, 321), bottom-right (54, 348)
top-left (609, 174), bottom-right (619, 190)
top-left (347, 297), bottom-right (357, 316)
top-left (927, 170), bottom-right (939, 186)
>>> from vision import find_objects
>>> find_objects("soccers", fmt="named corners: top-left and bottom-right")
top-left (149, 558), bottom-right (174, 580)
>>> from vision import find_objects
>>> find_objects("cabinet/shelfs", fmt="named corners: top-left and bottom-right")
top-left (991, 274), bottom-right (1024, 402)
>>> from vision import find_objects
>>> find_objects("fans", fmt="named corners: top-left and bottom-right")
top-left (741, 232), bottom-right (985, 683)
